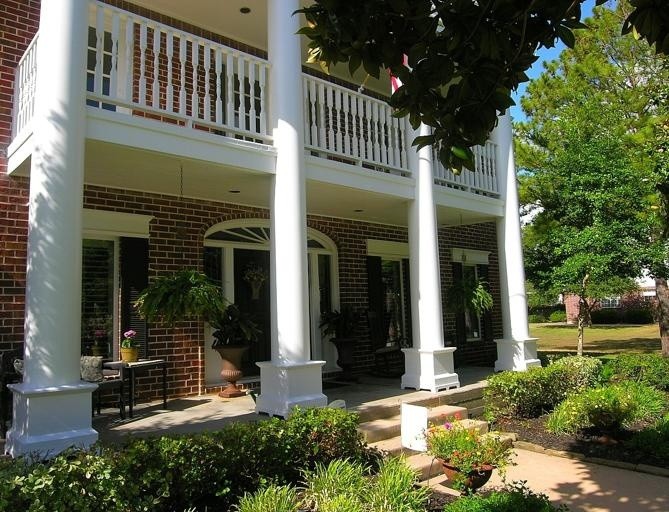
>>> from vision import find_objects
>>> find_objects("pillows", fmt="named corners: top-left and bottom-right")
top-left (78, 354), bottom-right (105, 381)
top-left (11, 355), bottom-right (25, 375)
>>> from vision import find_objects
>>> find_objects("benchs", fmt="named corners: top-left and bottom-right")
top-left (0, 345), bottom-right (126, 438)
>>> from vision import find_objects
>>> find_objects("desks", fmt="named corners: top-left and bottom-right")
top-left (102, 357), bottom-right (170, 420)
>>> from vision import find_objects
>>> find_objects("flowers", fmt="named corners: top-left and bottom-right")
top-left (414, 408), bottom-right (520, 490)
top-left (120, 329), bottom-right (139, 347)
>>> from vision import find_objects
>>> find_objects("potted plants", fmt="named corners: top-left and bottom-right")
top-left (318, 306), bottom-right (363, 381)
top-left (207, 303), bottom-right (255, 398)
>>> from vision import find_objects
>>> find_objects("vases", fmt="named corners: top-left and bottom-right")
top-left (433, 455), bottom-right (497, 490)
top-left (117, 344), bottom-right (140, 363)
